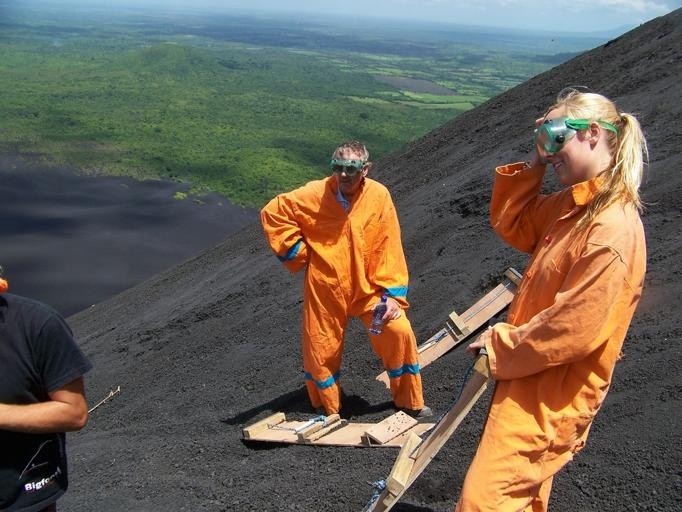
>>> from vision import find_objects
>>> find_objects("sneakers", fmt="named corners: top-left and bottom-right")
top-left (393, 405), bottom-right (433, 419)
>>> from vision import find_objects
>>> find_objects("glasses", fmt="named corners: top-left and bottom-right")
top-left (329, 160), bottom-right (362, 176)
top-left (533, 116), bottom-right (577, 163)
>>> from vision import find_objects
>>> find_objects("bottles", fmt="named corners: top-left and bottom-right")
top-left (368, 295), bottom-right (388, 335)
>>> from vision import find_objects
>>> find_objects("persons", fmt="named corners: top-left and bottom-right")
top-left (0, 292), bottom-right (92, 512)
top-left (259, 141), bottom-right (434, 416)
top-left (455, 86), bottom-right (648, 512)
